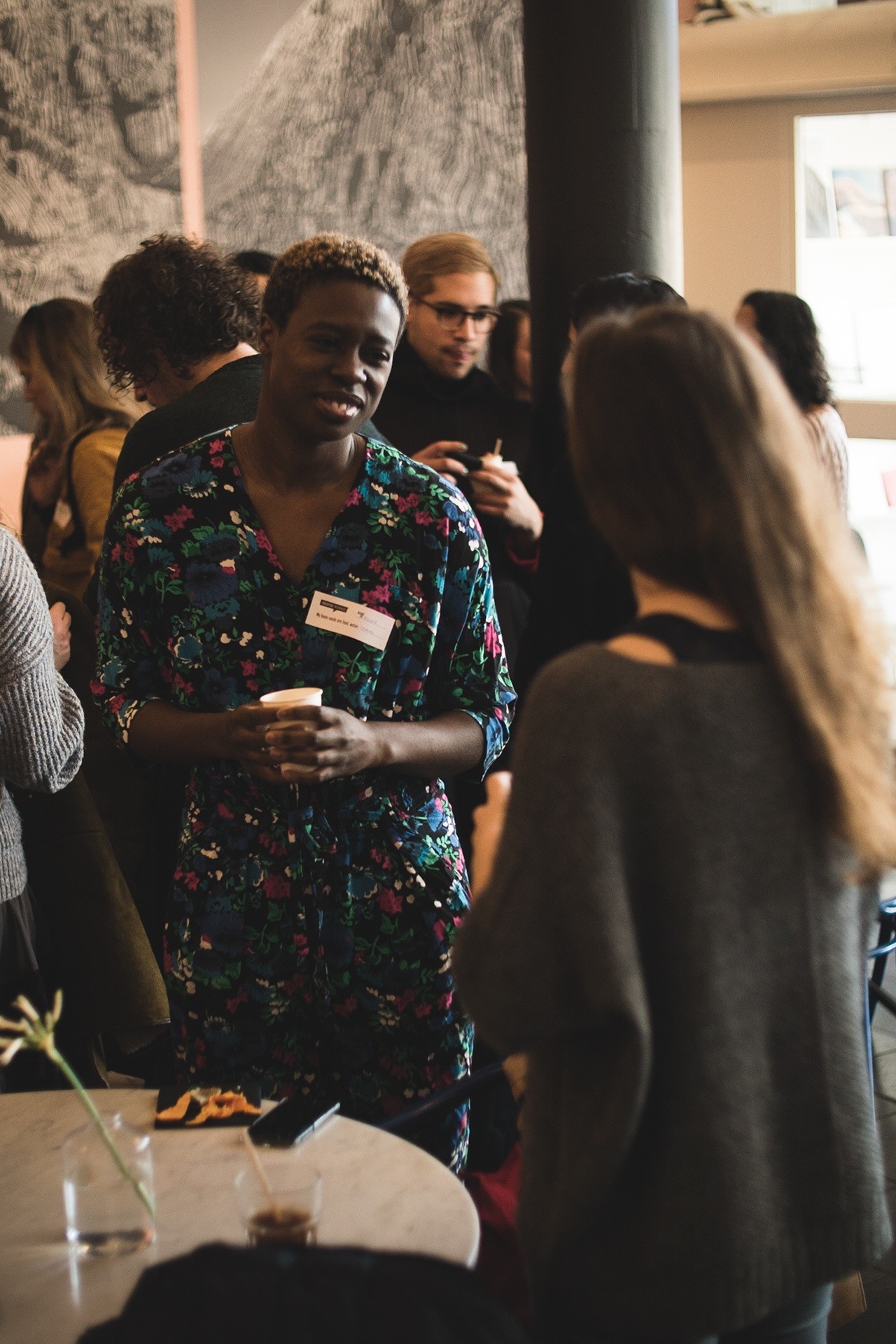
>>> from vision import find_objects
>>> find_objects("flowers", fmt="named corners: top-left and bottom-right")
top-left (0, 990), bottom-right (153, 1217)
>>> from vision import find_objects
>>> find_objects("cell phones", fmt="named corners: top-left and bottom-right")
top-left (247, 1091), bottom-right (341, 1150)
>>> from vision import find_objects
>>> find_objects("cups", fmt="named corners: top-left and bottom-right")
top-left (260, 687), bottom-right (324, 774)
top-left (236, 1164), bottom-right (325, 1248)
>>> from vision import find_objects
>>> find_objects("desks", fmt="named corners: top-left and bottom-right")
top-left (0, 1090), bottom-right (483, 1344)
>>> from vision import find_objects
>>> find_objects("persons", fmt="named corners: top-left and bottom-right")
top-left (450, 307), bottom-right (896, 1344)
top-left (515, 272), bottom-right (690, 692)
top-left (89, 233), bottom-right (517, 1184)
top-left (93, 233), bottom-right (265, 501)
top-left (736, 290), bottom-right (849, 516)
top-left (0, 527), bottom-right (84, 1093)
top-left (10, 297), bottom-right (135, 596)
top-left (369, 231), bottom-right (545, 661)
top-left (486, 299), bottom-right (533, 403)
top-left (230, 250), bottom-right (278, 308)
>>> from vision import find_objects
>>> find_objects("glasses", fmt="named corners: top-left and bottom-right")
top-left (406, 288), bottom-right (503, 336)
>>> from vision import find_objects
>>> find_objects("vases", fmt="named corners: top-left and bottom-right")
top-left (61, 1111), bottom-right (156, 1258)
